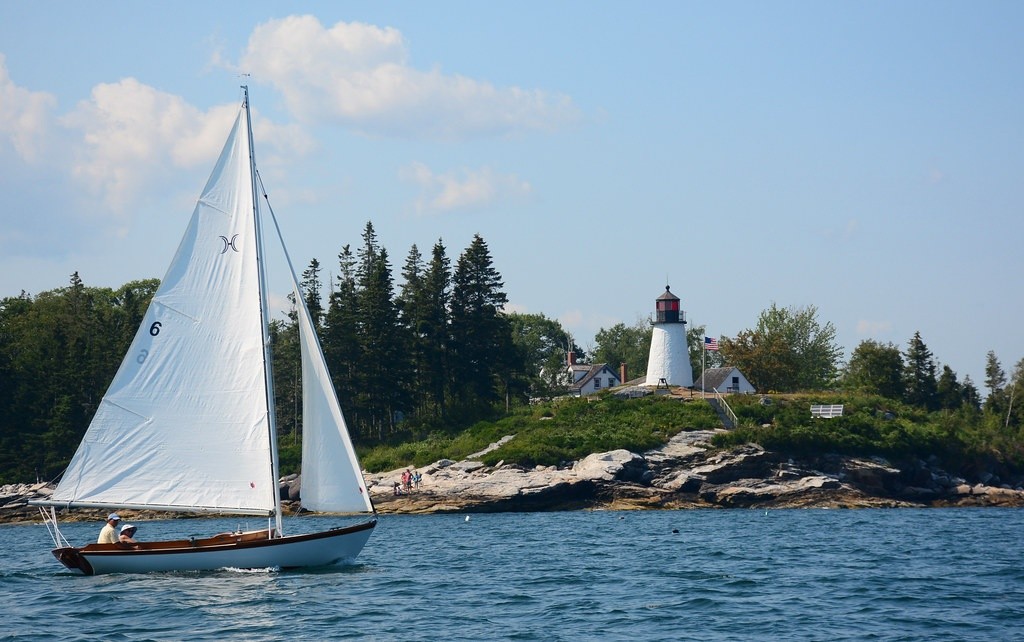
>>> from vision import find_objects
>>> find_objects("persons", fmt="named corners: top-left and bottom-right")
top-left (413, 471), bottom-right (420, 489)
top-left (404, 469), bottom-right (414, 492)
top-left (400, 472), bottom-right (407, 491)
top-left (96, 513), bottom-right (135, 550)
top-left (118, 524), bottom-right (137, 543)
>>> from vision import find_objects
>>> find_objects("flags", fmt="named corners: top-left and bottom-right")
top-left (705, 337), bottom-right (718, 350)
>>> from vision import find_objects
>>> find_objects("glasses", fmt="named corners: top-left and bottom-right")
top-left (127, 530), bottom-right (133, 532)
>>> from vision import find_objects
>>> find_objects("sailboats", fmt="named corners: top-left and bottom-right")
top-left (3, 83), bottom-right (379, 575)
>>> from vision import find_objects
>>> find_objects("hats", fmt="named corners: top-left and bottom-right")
top-left (107, 513), bottom-right (121, 520)
top-left (120, 524), bottom-right (137, 538)
